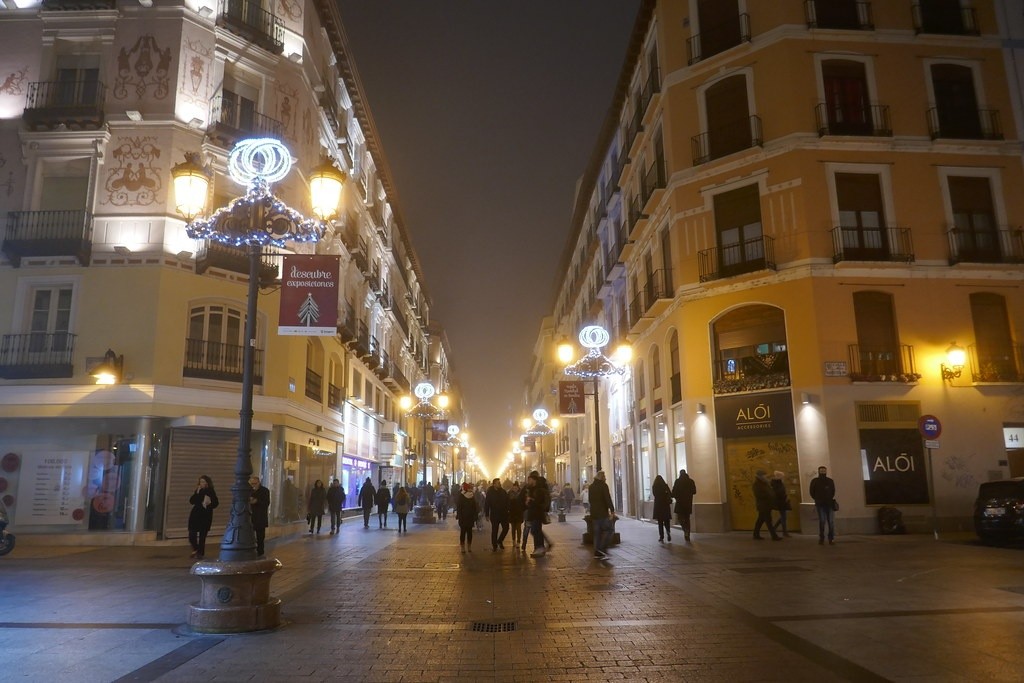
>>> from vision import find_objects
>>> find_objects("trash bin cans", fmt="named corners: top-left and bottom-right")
top-left (879, 507), bottom-right (902, 535)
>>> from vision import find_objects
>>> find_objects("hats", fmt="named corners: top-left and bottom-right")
top-left (366, 477), bottom-right (371, 482)
top-left (440, 485), bottom-right (445, 489)
top-left (755, 470), bottom-right (767, 476)
top-left (462, 482), bottom-right (472, 489)
top-left (380, 480), bottom-right (387, 485)
top-left (527, 471), bottom-right (541, 479)
top-left (774, 471), bottom-right (784, 479)
top-left (594, 471), bottom-right (607, 480)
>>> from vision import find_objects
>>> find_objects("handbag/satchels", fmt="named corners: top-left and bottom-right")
top-left (665, 484), bottom-right (673, 504)
top-left (832, 499), bottom-right (839, 511)
top-left (542, 512), bottom-right (551, 525)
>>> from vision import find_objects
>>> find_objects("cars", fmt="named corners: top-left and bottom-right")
top-left (972, 476), bottom-right (1024, 543)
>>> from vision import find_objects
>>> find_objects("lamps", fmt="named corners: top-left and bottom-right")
top-left (350, 395), bottom-right (362, 401)
top-left (800, 392), bottom-right (809, 404)
top-left (658, 415), bottom-right (665, 424)
top-left (363, 404), bottom-right (374, 410)
top-left (374, 412), bottom-right (384, 417)
top-left (643, 422), bottom-right (648, 431)
top-left (88, 348), bottom-right (124, 382)
top-left (941, 342), bottom-right (966, 381)
top-left (696, 403), bottom-right (704, 414)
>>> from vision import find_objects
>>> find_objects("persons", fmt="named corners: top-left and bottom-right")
top-left (357, 478), bottom-right (376, 530)
top-left (617, 479), bottom-right (622, 503)
top-left (589, 471), bottom-right (616, 560)
top-left (652, 470), bottom-right (696, 545)
top-left (395, 487), bottom-right (411, 533)
top-left (518, 471), bottom-right (553, 557)
top-left (456, 482), bottom-right (479, 554)
top-left (308, 480), bottom-right (326, 533)
top-left (508, 481), bottom-right (522, 548)
top-left (809, 466), bottom-right (838, 546)
top-left (578, 483), bottom-right (589, 514)
top-left (249, 476), bottom-right (270, 557)
top-left (558, 482), bottom-right (576, 513)
top-left (327, 479), bottom-right (346, 535)
top-left (484, 479), bottom-right (509, 552)
top-left (752, 470), bottom-right (793, 541)
top-left (389, 478), bottom-right (559, 530)
top-left (376, 480), bottom-right (392, 529)
top-left (188, 475), bottom-right (219, 559)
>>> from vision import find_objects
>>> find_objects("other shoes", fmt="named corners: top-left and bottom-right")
top-left (256, 547), bottom-right (265, 557)
top-left (818, 538), bottom-right (835, 545)
top-left (364, 526), bottom-right (369, 529)
top-left (317, 531), bottom-right (320, 534)
top-left (378, 523), bottom-right (408, 534)
top-left (491, 542), bottom-right (552, 556)
top-left (783, 534), bottom-right (792, 538)
top-left (684, 533), bottom-right (691, 544)
top-left (593, 548), bottom-right (613, 559)
top-left (191, 549), bottom-right (204, 559)
top-left (468, 546), bottom-right (472, 552)
top-left (658, 535), bottom-right (672, 543)
top-left (752, 535), bottom-right (766, 539)
top-left (437, 519), bottom-right (448, 524)
top-left (330, 530), bottom-right (340, 535)
top-left (772, 536), bottom-right (784, 540)
top-left (309, 530), bottom-right (314, 534)
top-left (461, 545), bottom-right (466, 553)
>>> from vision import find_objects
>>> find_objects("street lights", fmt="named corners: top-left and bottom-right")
top-left (169, 137), bottom-right (349, 631)
top-left (446, 425), bottom-right (489, 485)
top-left (400, 382), bottom-right (451, 524)
top-left (557, 325), bottom-right (635, 544)
top-left (500, 408), bottom-right (561, 478)
